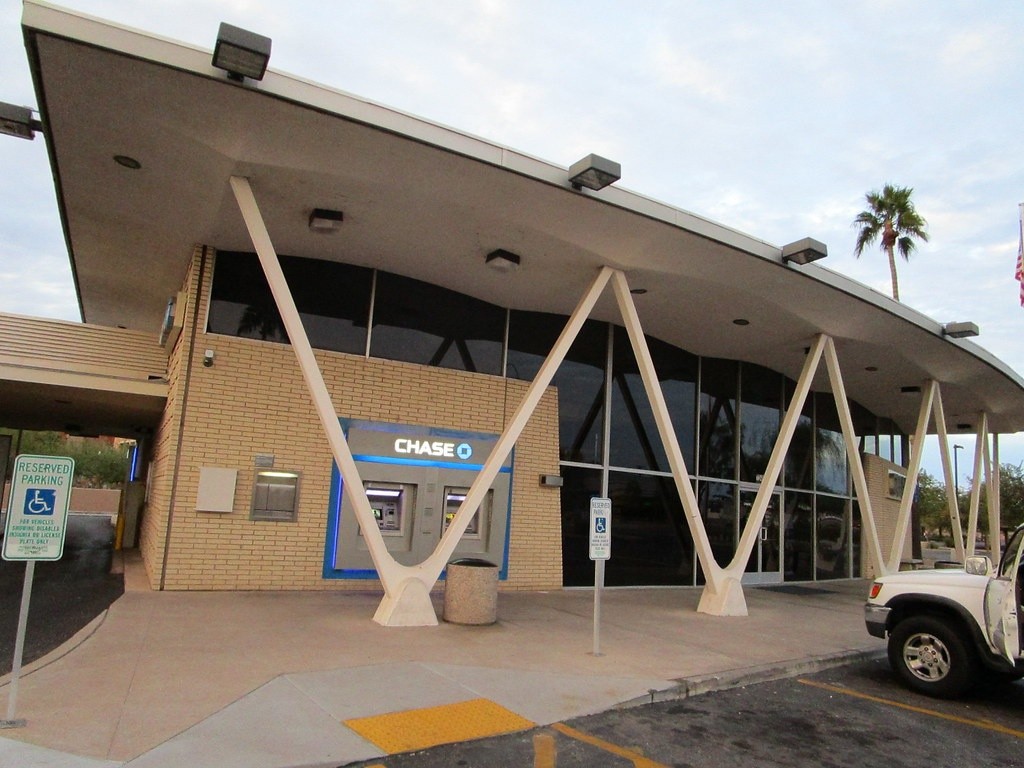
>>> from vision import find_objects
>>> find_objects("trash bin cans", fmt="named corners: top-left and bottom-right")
top-left (934, 561), bottom-right (964, 569)
top-left (443, 557), bottom-right (501, 626)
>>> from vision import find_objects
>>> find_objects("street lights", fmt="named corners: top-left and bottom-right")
top-left (954, 445), bottom-right (964, 502)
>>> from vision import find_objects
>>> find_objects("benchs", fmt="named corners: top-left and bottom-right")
top-left (900, 558), bottom-right (925, 570)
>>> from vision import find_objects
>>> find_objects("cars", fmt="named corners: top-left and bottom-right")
top-left (705, 490), bottom-right (862, 581)
top-left (863, 522), bottom-right (1024, 699)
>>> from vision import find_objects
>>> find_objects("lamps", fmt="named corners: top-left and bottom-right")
top-left (210, 21), bottom-right (273, 85)
top-left (568, 154), bottom-right (622, 193)
top-left (956, 424), bottom-right (971, 433)
top-left (484, 249), bottom-right (521, 275)
top-left (308, 207), bottom-right (344, 235)
top-left (803, 346), bottom-right (825, 360)
top-left (900, 386), bottom-right (921, 398)
top-left (943, 321), bottom-right (979, 338)
top-left (146, 375), bottom-right (171, 382)
top-left (0, 101), bottom-right (43, 141)
top-left (780, 236), bottom-right (828, 267)
